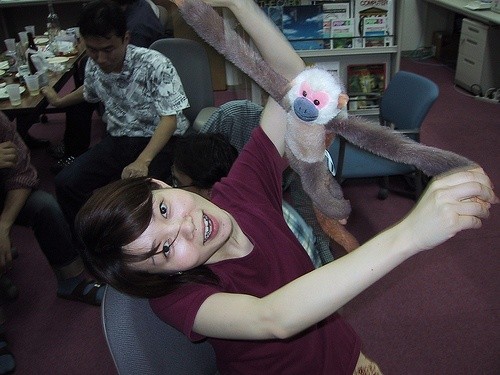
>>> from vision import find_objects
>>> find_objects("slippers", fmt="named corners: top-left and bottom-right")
top-left (57, 278), bottom-right (104, 306)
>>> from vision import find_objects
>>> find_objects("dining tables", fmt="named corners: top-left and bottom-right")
top-left (0, 36), bottom-right (89, 124)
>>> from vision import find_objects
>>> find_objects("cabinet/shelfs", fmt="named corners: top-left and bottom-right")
top-left (241, 0), bottom-right (404, 129)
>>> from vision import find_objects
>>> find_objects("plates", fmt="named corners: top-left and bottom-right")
top-left (34, 38), bottom-right (49, 44)
top-left (47, 56), bottom-right (69, 64)
top-left (0, 87), bottom-right (26, 98)
top-left (0, 60), bottom-right (9, 75)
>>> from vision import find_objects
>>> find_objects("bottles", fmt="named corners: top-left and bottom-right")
top-left (27, 32), bottom-right (39, 74)
top-left (46, 4), bottom-right (60, 31)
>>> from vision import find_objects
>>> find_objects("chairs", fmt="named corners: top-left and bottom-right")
top-left (101, 283), bottom-right (218, 375)
top-left (327, 71), bottom-right (439, 187)
top-left (149, 38), bottom-right (218, 132)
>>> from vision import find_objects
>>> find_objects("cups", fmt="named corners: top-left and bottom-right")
top-left (25, 75), bottom-right (40, 96)
top-left (4, 39), bottom-right (16, 52)
top-left (6, 84), bottom-right (21, 106)
top-left (25, 26), bottom-right (35, 38)
top-left (18, 32), bottom-right (28, 44)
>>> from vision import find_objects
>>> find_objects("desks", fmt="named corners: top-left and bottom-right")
top-left (425, 0), bottom-right (500, 27)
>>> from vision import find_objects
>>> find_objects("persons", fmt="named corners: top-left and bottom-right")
top-left (77, 0), bottom-right (500, 375)
top-left (41, 0), bottom-right (190, 244)
top-left (0, 111), bottom-right (107, 375)
top-left (120, 0), bottom-right (166, 50)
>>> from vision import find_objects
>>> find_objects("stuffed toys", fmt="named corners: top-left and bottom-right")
top-left (172, 0), bottom-right (491, 252)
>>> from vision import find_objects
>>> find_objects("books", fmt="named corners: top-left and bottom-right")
top-left (255, 0), bottom-right (394, 111)
